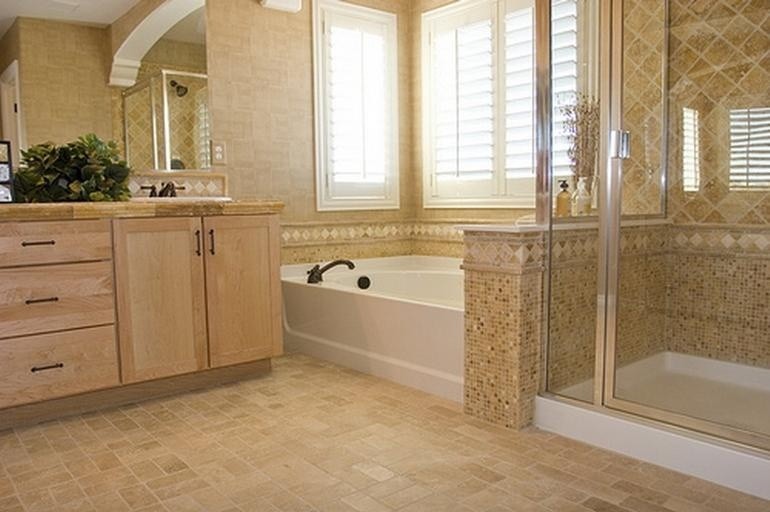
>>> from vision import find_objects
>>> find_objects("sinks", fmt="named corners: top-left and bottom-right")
top-left (130, 195), bottom-right (233, 202)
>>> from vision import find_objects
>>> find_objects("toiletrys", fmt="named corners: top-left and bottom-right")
top-left (554, 182), bottom-right (571, 216)
top-left (555, 179), bottom-right (571, 217)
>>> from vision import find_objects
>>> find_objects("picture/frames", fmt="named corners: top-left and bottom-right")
top-left (0, 141), bottom-right (16, 203)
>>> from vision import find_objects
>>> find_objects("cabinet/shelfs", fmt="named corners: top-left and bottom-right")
top-left (112, 214), bottom-right (283, 403)
top-left (0, 217), bottom-right (122, 431)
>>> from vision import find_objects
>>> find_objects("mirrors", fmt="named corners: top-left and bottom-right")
top-left (0, 0), bottom-right (210, 172)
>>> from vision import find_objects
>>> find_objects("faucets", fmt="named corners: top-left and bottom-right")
top-left (306, 256), bottom-right (355, 284)
top-left (158, 180), bottom-right (174, 196)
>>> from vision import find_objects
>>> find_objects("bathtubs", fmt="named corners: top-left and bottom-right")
top-left (279, 254), bottom-right (463, 402)
